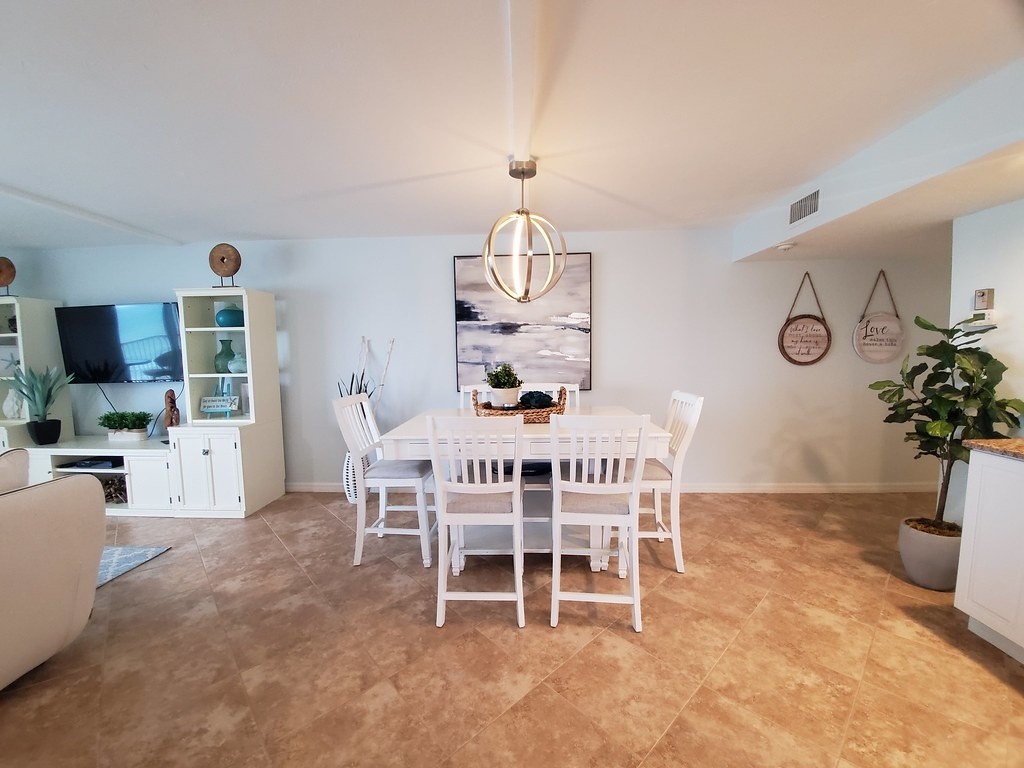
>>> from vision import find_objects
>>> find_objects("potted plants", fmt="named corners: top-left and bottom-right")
top-left (472, 386), bottom-right (566, 424)
top-left (868, 312), bottom-right (1024, 591)
top-left (12, 367), bottom-right (73, 443)
top-left (484, 365), bottom-right (521, 407)
top-left (100, 408), bottom-right (152, 440)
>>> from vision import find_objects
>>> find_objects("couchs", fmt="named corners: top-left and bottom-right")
top-left (0, 447), bottom-right (107, 693)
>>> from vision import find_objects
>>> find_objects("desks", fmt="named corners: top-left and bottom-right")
top-left (379, 407), bottom-right (672, 578)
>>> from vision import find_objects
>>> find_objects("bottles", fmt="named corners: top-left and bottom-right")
top-left (227, 352), bottom-right (247, 373)
top-left (2, 388), bottom-right (22, 418)
top-left (216, 303), bottom-right (244, 327)
top-left (214, 339), bottom-right (235, 373)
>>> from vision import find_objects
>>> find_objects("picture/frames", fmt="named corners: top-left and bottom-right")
top-left (777, 314), bottom-right (833, 366)
top-left (852, 313), bottom-right (907, 365)
top-left (453, 253), bottom-right (591, 392)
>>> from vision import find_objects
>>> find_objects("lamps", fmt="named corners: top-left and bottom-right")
top-left (482, 151), bottom-right (567, 301)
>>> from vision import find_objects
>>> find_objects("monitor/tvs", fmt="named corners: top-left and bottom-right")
top-left (54, 302), bottom-right (184, 384)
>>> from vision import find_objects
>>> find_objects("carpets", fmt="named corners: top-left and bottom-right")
top-left (96, 547), bottom-right (172, 591)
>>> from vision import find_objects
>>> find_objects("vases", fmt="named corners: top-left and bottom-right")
top-left (343, 450), bottom-right (371, 505)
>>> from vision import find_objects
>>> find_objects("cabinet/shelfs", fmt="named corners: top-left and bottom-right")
top-left (175, 290), bottom-right (255, 423)
top-left (955, 449), bottom-right (1023, 666)
top-left (24, 447), bottom-right (171, 520)
top-left (0, 296), bottom-right (31, 424)
top-left (168, 429), bottom-right (247, 519)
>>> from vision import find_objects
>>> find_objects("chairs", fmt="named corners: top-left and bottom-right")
top-left (425, 414), bottom-right (527, 630)
top-left (518, 380), bottom-right (579, 419)
top-left (333, 392), bottom-right (437, 568)
top-left (604, 389), bottom-right (704, 573)
top-left (460, 384), bottom-right (521, 409)
top-left (546, 413), bottom-right (651, 633)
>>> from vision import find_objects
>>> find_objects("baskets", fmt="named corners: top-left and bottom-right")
top-left (471, 386), bottom-right (567, 423)
